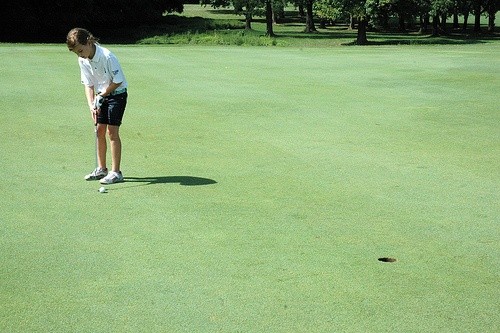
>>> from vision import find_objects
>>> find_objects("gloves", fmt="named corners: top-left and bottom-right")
top-left (92, 95), bottom-right (104, 109)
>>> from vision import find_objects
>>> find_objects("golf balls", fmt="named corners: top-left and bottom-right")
top-left (99, 187), bottom-right (105, 193)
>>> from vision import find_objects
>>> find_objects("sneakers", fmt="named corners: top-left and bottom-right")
top-left (100, 171), bottom-right (123, 183)
top-left (84, 168), bottom-right (108, 180)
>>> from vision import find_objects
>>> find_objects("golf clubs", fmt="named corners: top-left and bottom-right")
top-left (89, 100), bottom-right (104, 179)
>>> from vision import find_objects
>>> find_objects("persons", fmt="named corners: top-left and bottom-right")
top-left (66, 28), bottom-right (127, 184)
